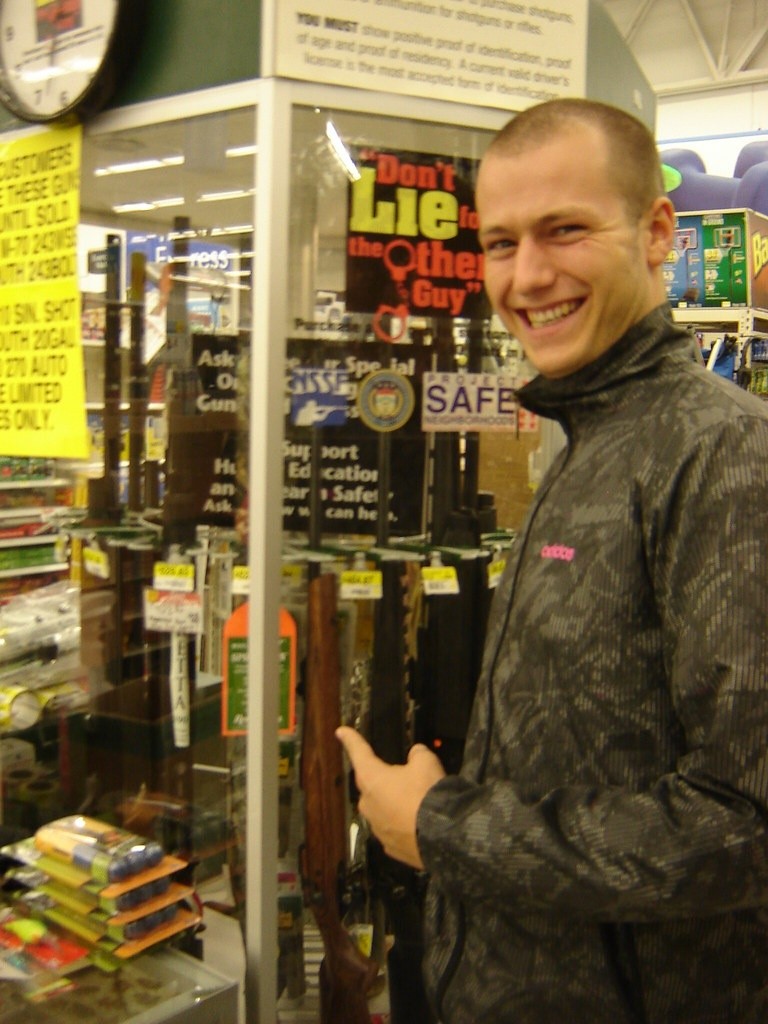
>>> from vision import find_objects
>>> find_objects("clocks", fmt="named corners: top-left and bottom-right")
top-left (0, 0), bottom-right (148, 123)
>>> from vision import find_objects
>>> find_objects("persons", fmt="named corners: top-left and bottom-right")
top-left (334, 98), bottom-right (767, 1024)
